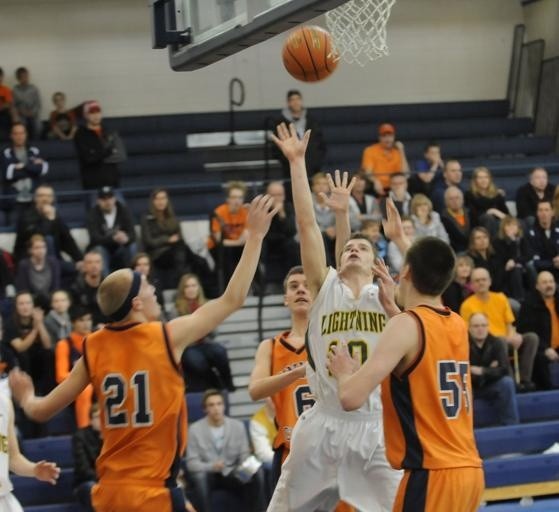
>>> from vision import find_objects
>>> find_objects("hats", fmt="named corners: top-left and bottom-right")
top-left (98, 186), bottom-right (115, 197)
top-left (82, 100), bottom-right (101, 113)
top-left (68, 303), bottom-right (92, 320)
top-left (379, 123), bottom-right (395, 135)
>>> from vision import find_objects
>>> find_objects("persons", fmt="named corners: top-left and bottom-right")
top-left (267, 87), bottom-right (325, 182)
top-left (132, 253), bottom-right (165, 314)
top-left (261, 186), bottom-right (305, 270)
top-left (265, 119), bottom-right (405, 512)
top-left (72, 402), bottom-right (104, 511)
top-left (8, 192), bottom-right (281, 511)
top-left (249, 391), bottom-right (290, 501)
top-left (249, 267), bottom-right (355, 512)
top-left (320, 170), bottom-right (413, 512)
top-left (183, 390), bottom-right (264, 512)
top-left (208, 179), bottom-right (266, 299)
top-left (167, 273), bottom-right (234, 393)
top-left (0, 341), bottom-right (61, 512)
top-left (309, 169), bottom-right (341, 267)
top-left (324, 236), bottom-right (485, 511)
top-left (140, 188), bottom-right (211, 301)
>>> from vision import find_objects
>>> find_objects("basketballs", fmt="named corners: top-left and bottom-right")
top-left (282, 26), bottom-right (338, 81)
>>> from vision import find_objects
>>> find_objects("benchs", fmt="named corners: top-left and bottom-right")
top-left (473, 390), bottom-right (558, 512)
top-left (8, 403), bottom-right (76, 507)
top-left (1, 121), bottom-right (557, 233)
top-left (187, 296), bottom-right (291, 428)
